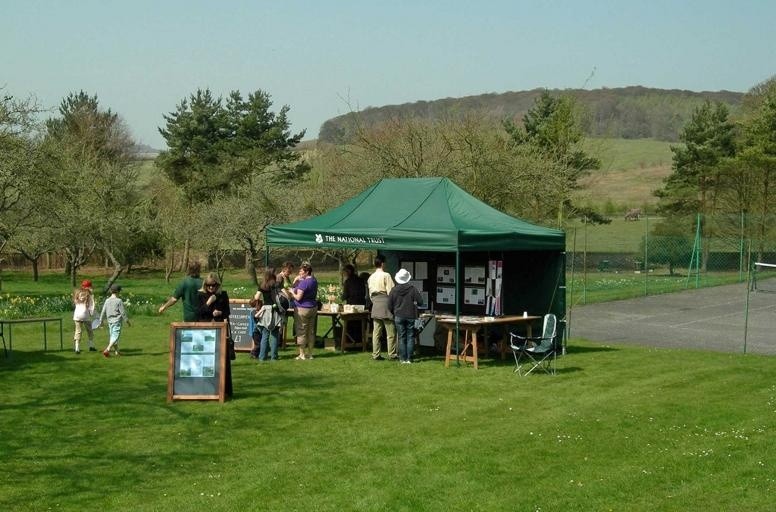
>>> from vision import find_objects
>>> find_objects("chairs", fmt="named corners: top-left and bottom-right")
top-left (508, 314), bottom-right (560, 377)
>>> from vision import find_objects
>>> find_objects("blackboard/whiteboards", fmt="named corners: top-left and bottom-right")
top-left (223, 299), bottom-right (256, 352)
top-left (168, 321), bottom-right (227, 400)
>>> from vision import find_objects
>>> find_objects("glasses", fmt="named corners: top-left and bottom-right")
top-left (206, 283), bottom-right (217, 287)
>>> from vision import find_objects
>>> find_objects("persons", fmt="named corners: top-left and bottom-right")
top-left (159, 260), bottom-right (204, 322)
top-left (248, 253), bottom-right (423, 364)
top-left (99, 283), bottom-right (131, 357)
top-left (196, 271), bottom-right (235, 403)
top-left (73, 278), bottom-right (97, 354)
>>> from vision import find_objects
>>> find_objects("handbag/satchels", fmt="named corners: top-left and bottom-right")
top-left (226, 336), bottom-right (235, 360)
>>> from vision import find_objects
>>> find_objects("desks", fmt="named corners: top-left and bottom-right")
top-left (0, 315), bottom-right (63, 353)
top-left (438, 316), bottom-right (540, 370)
top-left (282, 306), bottom-right (337, 352)
top-left (337, 311), bottom-right (433, 354)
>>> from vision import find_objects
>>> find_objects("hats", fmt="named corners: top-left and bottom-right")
top-left (111, 285), bottom-right (123, 292)
top-left (82, 280), bottom-right (92, 288)
top-left (395, 268), bottom-right (412, 284)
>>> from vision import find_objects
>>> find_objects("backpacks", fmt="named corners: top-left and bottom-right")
top-left (270, 288), bottom-right (289, 314)
